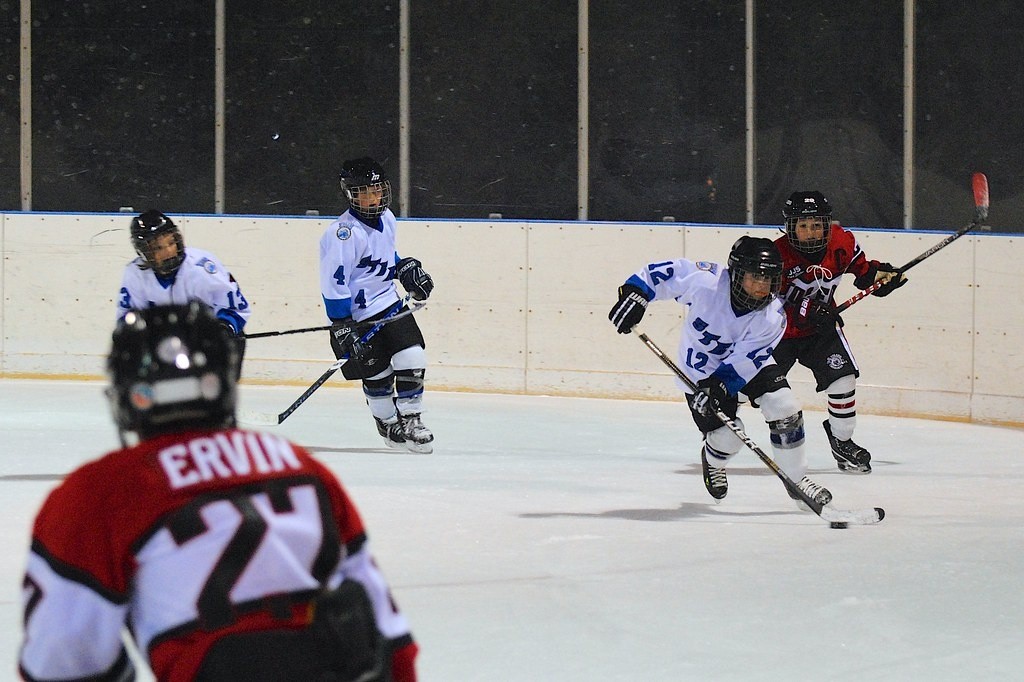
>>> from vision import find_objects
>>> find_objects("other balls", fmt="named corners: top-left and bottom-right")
top-left (830, 521), bottom-right (848, 530)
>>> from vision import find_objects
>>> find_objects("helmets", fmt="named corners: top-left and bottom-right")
top-left (130, 209), bottom-right (190, 276)
top-left (727, 235), bottom-right (782, 317)
top-left (340, 157), bottom-right (392, 220)
top-left (104, 299), bottom-right (244, 446)
top-left (782, 190), bottom-right (837, 263)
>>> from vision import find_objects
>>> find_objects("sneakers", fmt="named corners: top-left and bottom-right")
top-left (784, 477), bottom-right (834, 511)
top-left (823, 419), bottom-right (871, 475)
top-left (702, 445), bottom-right (729, 504)
top-left (392, 396), bottom-right (434, 454)
top-left (374, 411), bottom-right (407, 452)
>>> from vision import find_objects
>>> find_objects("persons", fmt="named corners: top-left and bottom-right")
top-left (18, 305), bottom-right (418, 682)
top-left (609, 236), bottom-right (832, 512)
top-left (767, 190), bottom-right (906, 475)
top-left (118, 209), bottom-right (249, 429)
top-left (320, 158), bottom-right (435, 454)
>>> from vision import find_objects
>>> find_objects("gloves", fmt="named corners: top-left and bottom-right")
top-left (608, 285), bottom-right (650, 334)
top-left (853, 261), bottom-right (909, 297)
top-left (396, 258), bottom-right (434, 300)
top-left (690, 374), bottom-right (728, 422)
top-left (329, 316), bottom-right (372, 363)
top-left (791, 296), bottom-right (845, 339)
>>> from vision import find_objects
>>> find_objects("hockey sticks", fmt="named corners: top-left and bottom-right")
top-left (832, 172), bottom-right (991, 315)
top-left (630, 323), bottom-right (885, 525)
top-left (233, 291), bottom-right (417, 427)
top-left (240, 301), bottom-right (428, 340)
